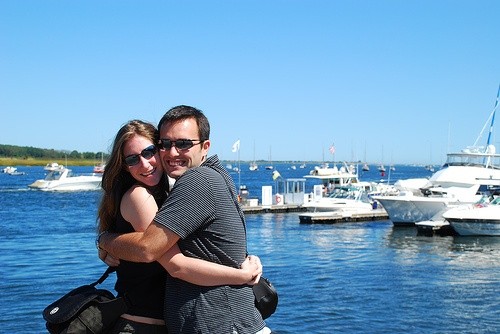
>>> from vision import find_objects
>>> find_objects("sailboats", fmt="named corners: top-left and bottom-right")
top-left (378, 142), bottom-right (386, 171)
top-left (361, 140), bottom-right (369, 171)
top-left (249, 135), bottom-right (257, 170)
top-left (265, 143), bottom-right (273, 169)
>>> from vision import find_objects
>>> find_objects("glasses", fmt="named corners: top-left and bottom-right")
top-left (158, 139), bottom-right (208, 151)
top-left (124, 145), bottom-right (157, 166)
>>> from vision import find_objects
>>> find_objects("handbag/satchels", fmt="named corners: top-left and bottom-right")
top-left (253, 277), bottom-right (279, 320)
top-left (42, 285), bottom-right (129, 334)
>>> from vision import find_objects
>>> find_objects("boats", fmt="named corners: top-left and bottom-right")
top-left (226, 163), bottom-right (241, 172)
top-left (1, 167), bottom-right (24, 175)
top-left (299, 83), bottom-right (500, 236)
top-left (27, 153), bottom-right (106, 192)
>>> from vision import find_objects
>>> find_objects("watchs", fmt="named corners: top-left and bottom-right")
top-left (96, 230), bottom-right (111, 252)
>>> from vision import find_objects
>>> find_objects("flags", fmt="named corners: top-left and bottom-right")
top-left (329, 144), bottom-right (336, 154)
top-left (232, 140), bottom-right (240, 152)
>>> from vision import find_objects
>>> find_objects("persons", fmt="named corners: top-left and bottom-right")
top-left (99, 120), bottom-right (263, 334)
top-left (96, 105), bottom-right (272, 334)
top-left (323, 180), bottom-right (341, 196)
